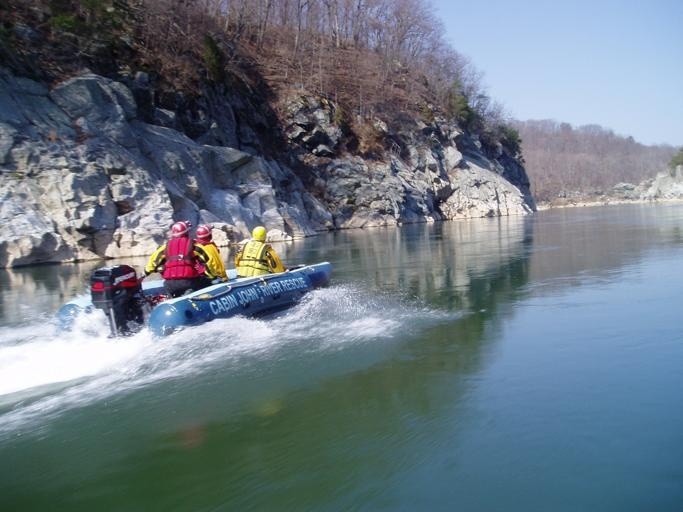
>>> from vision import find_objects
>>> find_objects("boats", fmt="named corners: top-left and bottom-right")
top-left (50, 258), bottom-right (338, 342)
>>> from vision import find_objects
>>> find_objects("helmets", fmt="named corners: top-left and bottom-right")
top-left (195, 224), bottom-right (214, 238)
top-left (252, 226), bottom-right (267, 241)
top-left (172, 220), bottom-right (192, 236)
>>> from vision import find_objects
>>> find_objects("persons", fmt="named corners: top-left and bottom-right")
top-left (140, 219), bottom-right (211, 300)
top-left (232, 225), bottom-right (285, 280)
top-left (190, 222), bottom-right (228, 288)
